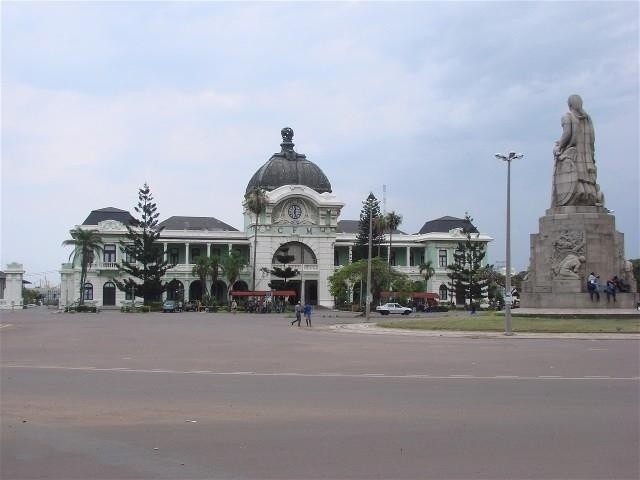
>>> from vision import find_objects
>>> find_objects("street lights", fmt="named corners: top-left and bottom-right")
top-left (493, 151), bottom-right (524, 336)
top-left (361, 197), bottom-right (382, 320)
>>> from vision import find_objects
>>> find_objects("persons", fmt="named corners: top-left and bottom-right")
top-left (559, 255), bottom-right (585, 280)
top-left (412, 302), bottom-right (416, 312)
top-left (244, 298), bottom-right (285, 313)
top-left (587, 272), bottom-right (601, 303)
top-left (292, 301), bottom-right (301, 327)
top-left (178, 300), bottom-right (183, 312)
top-left (553, 95), bottom-right (597, 205)
top-left (607, 276), bottom-right (631, 304)
top-left (304, 302), bottom-right (312, 327)
top-left (231, 299), bottom-right (237, 312)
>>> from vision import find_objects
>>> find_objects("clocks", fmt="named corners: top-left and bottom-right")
top-left (288, 205), bottom-right (302, 219)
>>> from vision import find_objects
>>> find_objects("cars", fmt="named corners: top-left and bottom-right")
top-left (375, 303), bottom-right (412, 316)
top-left (162, 299), bottom-right (198, 313)
top-left (64, 301), bottom-right (95, 312)
